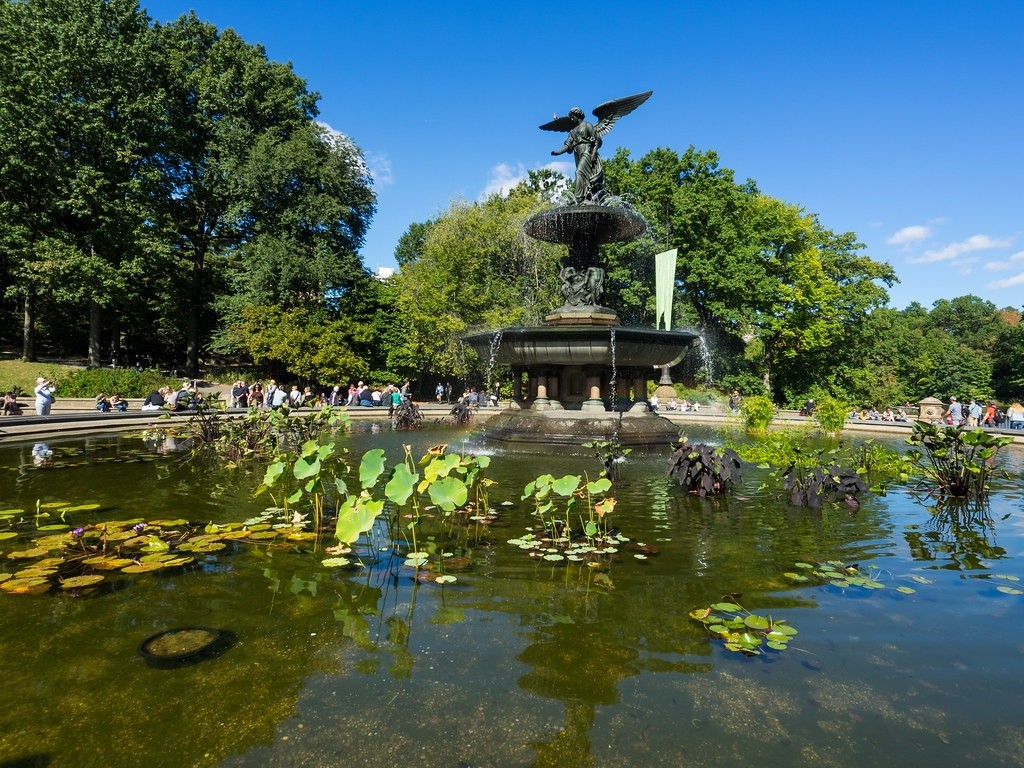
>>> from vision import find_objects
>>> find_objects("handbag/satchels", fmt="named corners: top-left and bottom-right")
top-left (50, 396), bottom-right (56, 403)
top-left (289, 398), bottom-right (294, 406)
top-left (306, 395), bottom-right (315, 401)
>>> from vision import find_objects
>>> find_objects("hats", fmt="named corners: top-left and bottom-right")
top-left (949, 396), bottom-right (956, 401)
top-left (988, 400), bottom-right (996, 404)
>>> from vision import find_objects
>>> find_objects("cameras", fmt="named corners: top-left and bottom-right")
top-left (49, 381), bottom-right (54, 384)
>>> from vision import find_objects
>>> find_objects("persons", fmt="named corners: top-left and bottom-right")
top-left (94, 374), bottom-right (500, 413)
top-left (551, 106), bottom-right (602, 205)
top-left (34, 377), bottom-right (55, 415)
top-left (651, 390), bottom-right (1024, 430)
top-left (4, 391), bottom-right (16, 415)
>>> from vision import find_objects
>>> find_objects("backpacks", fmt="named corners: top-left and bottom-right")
top-left (961, 404), bottom-right (970, 419)
top-left (989, 407), bottom-right (1006, 423)
